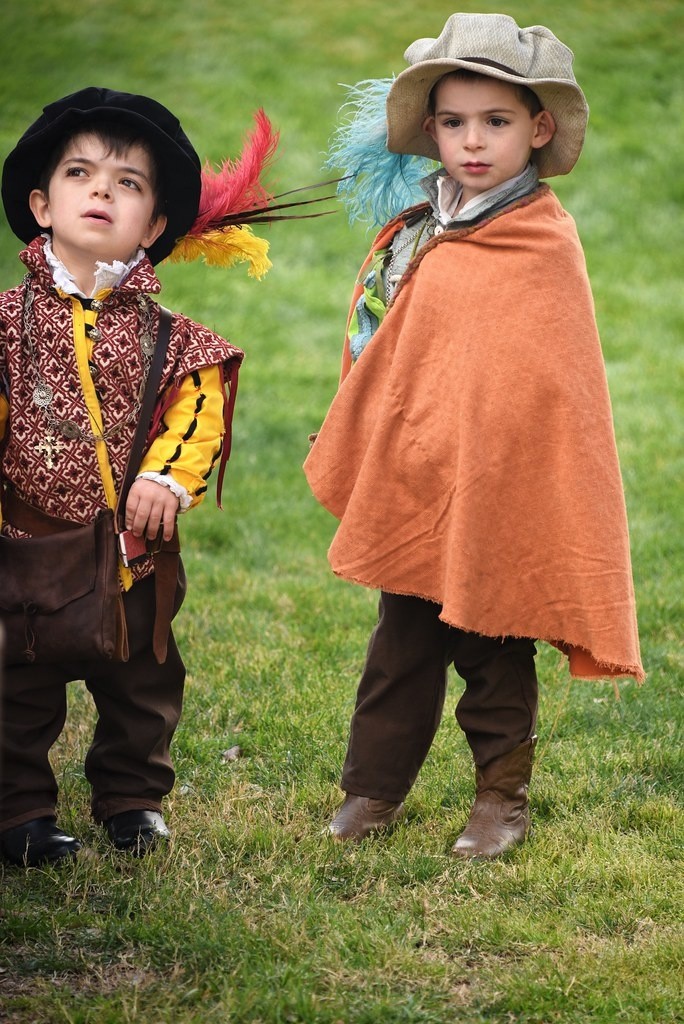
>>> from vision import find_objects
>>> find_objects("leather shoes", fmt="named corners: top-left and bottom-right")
top-left (451, 787), bottom-right (534, 860)
top-left (316, 793), bottom-right (408, 847)
top-left (0, 817), bottom-right (83, 868)
top-left (102, 809), bottom-right (172, 859)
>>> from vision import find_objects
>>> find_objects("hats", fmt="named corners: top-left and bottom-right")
top-left (317, 12), bottom-right (590, 242)
top-left (1, 86), bottom-right (358, 282)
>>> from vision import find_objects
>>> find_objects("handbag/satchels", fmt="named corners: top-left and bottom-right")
top-left (0, 508), bottom-right (130, 665)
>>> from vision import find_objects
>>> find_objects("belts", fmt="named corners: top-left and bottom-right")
top-left (1, 490), bottom-right (180, 665)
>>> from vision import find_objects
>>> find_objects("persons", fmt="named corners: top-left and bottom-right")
top-left (295, 12), bottom-right (644, 860)
top-left (0, 88), bottom-right (246, 871)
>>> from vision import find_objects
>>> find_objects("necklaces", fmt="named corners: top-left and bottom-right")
top-left (385, 218), bottom-right (428, 308)
top-left (22, 273), bottom-right (155, 439)
